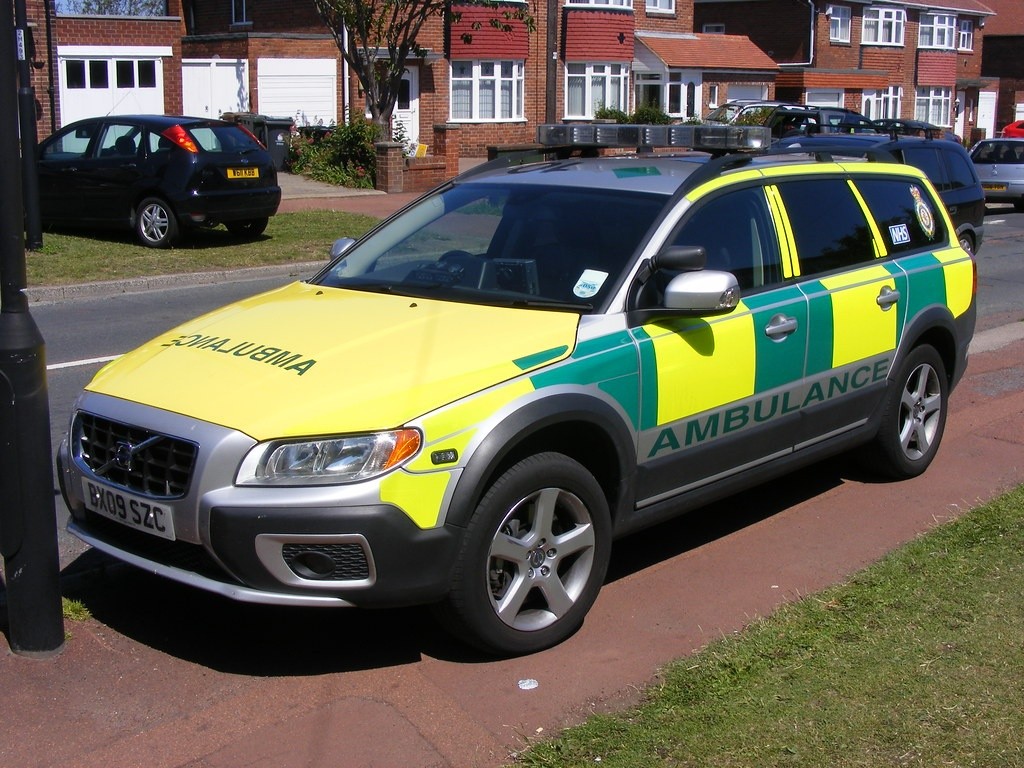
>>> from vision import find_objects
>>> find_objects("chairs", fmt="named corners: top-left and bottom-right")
top-left (103, 135), bottom-right (137, 156)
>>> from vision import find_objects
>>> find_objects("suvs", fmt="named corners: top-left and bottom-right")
top-left (762, 103), bottom-right (870, 135)
top-left (758, 127), bottom-right (987, 261)
top-left (701, 100), bottom-right (795, 126)
top-left (55, 123), bottom-right (977, 670)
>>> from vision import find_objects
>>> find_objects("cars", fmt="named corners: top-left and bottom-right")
top-left (966, 135), bottom-right (1024, 210)
top-left (35, 111), bottom-right (284, 245)
top-left (1001, 120), bottom-right (1024, 137)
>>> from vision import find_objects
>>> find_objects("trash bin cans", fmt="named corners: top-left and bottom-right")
top-left (257, 115), bottom-right (295, 173)
top-left (219, 111), bottom-right (258, 134)
